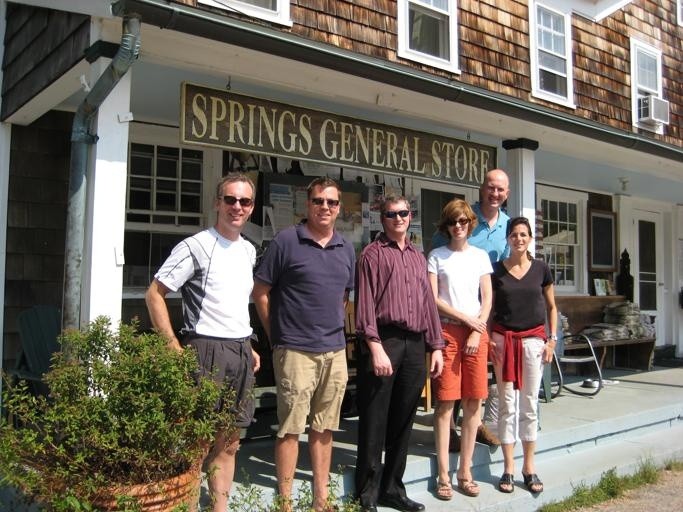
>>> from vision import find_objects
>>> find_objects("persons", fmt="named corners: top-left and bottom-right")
top-left (357, 193), bottom-right (444, 512)
top-left (146, 173), bottom-right (260, 512)
top-left (255, 177), bottom-right (358, 512)
top-left (432, 169), bottom-right (514, 453)
top-left (427, 197), bottom-right (493, 500)
top-left (491, 217), bottom-right (561, 494)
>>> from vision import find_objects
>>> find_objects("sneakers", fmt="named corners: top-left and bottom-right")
top-left (449, 429), bottom-right (460, 453)
top-left (477, 423), bottom-right (500, 446)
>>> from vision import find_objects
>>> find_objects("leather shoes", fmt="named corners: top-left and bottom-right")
top-left (359, 502), bottom-right (377, 512)
top-left (379, 496), bottom-right (425, 512)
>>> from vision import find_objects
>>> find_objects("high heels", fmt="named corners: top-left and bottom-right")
top-left (457, 478), bottom-right (480, 496)
top-left (499, 472), bottom-right (514, 492)
top-left (522, 471), bottom-right (543, 493)
top-left (437, 480), bottom-right (452, 500)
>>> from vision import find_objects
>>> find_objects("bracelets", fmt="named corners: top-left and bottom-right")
top-left (549, 334), bottom-right (559, 341)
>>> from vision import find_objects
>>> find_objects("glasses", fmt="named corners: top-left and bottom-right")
top-left (312, 198), bottom-right (339, 206)
top-left (448, 219), bottom-right (467, 226)
top-left (218, 195), bottom-right (254, 206)
top-left (383, 210), bottom-right (409, 218)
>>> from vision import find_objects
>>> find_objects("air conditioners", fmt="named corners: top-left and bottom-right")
top-left (637, 93), bottom-right (670, 126)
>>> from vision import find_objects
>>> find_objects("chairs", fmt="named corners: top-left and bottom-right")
top-left (8, 303), bottom-right (61, 402)
top-left (534, 333), bottom-right (605, 401)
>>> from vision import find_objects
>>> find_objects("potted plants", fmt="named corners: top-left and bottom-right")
top-left (0, 312), bottom-right (254, 510)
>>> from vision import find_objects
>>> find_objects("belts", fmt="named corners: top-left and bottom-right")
top-left (441, 317), bottom-right (462, 326)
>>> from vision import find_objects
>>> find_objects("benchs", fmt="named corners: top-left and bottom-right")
top-left (548, 296), bottom-right (658, 378)
top-left (246, 296), bottom-right (436, 440)
